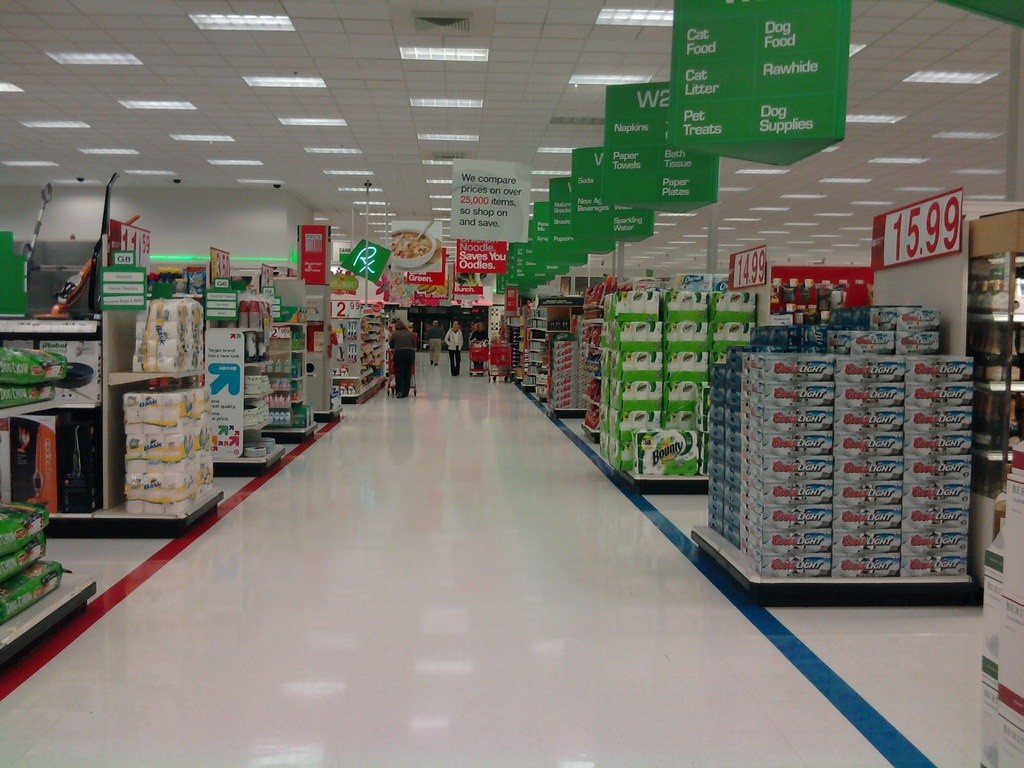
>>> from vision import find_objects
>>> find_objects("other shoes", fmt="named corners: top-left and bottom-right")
top-left (431, 360), bottom-right (433, 365)
top-left (434, 363), bottom-right (438, 365)
top-left (396, 392), bottom-right (402, 398)
top-left (452, 371), bottom-right (459, 376)
top-left (402, 391), bottom-right (408, 397)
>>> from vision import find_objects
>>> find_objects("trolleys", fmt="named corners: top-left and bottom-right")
top-left (469, 340), bottom-right (490, 376)
top-left (385, 348), bottom-right (418, 398)
top-left (488, 342), bottom-right (513, 385)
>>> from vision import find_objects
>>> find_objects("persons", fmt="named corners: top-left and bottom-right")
top-left (425, 320), bottom-right (446, 366)
top-left (389, 321), bottom-right (416, 398)
top-left (469, 322), bottom-right (489, 376)
top-left (444, 321), bottom-right (463, 376)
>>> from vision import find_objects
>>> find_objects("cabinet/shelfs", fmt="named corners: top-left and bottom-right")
top-left (967, 210), bottom-right (1024, 500)
top-left (582, 318), bottom-right (604, 444)
top-left (506, 304), bottom-right (547, 402)
top-left (0, 233), bottom-right (224, 538)
top-left (0, 398), bottom-right (97, 669)
top-left (146, 262), bottom-right (397, 476)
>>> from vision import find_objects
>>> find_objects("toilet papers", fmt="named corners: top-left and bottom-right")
top-left (123, 386), bottom-right (213, 514)
top-left (133, 299), bottom-right (203, 372)
top-left (600, 291), bottom-right (756, 477)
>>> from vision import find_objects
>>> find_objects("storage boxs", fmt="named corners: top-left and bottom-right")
top-left (980, 442), bottom-right (1024, 768)
top-left (0, 339), bottom-right (102, 513)
top-left (706, 306), bottom-right (973, 580)
top-left (553, 342), bottom-right (577, 408)
